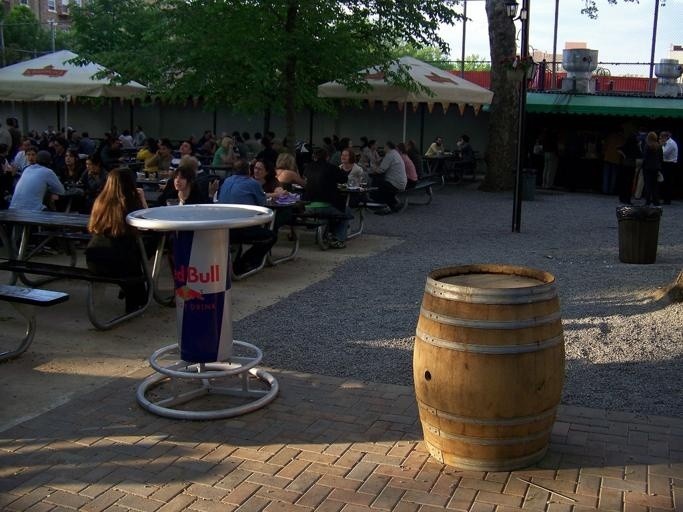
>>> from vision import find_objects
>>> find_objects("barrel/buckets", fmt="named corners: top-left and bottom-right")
top-left (412, 262), bottom-right (567, 472)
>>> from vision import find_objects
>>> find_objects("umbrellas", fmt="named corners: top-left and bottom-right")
top-left (0, 48), bottom-right (148, 139)
top-left (317, 56), bottom-right (495, 145)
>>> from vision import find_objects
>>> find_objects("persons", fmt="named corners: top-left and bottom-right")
top-left (1, 118), bottom-right (478, 319)
top-left (537, 59), bottom-right (551, 92)
top-left (634, 130), bottom-right (679, 207)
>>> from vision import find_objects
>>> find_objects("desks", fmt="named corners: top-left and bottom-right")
top-left (120, 202), bottom-right (283, 424)
top-left (1, 205), bottom-right (158, 327)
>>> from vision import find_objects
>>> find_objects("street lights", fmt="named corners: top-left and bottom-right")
top-left (503, 1), bottom-right (531, 230)
top-left (48, 17), bottom-right (56, 52)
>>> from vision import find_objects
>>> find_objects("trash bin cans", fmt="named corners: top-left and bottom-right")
top-left (616, 204), bottom-right (663, 264)
top-left (512, 168), bottom-right (538, 201)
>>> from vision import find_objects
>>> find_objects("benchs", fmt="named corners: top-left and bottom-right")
top-left (60, 137), bottom-right (481, 309)
top-left (1, 278), bottom-right (73, 360)
top-left (3, 255), bottom-right (133, 299)
top-left (28, 229), bottom-right (102, 253)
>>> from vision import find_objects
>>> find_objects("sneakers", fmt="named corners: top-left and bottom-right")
top-left (326, 232), bottom-right (345, 248)
top-left (396, 200), bottom-right (408, 215)
